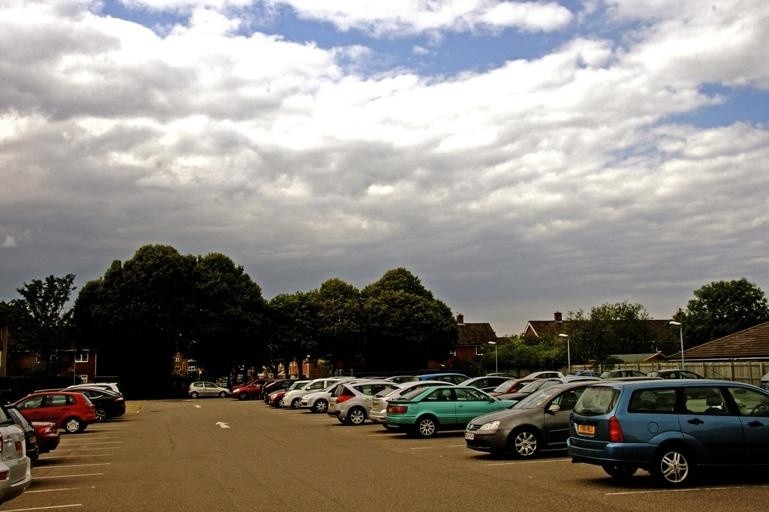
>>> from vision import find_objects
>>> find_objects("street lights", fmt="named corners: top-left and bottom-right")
top-left (488, 341), bottom-right (499, 374)
top-left (669, 320), bottom-right (687, 373)
top-left (559, 333), bottom-right (571, 375)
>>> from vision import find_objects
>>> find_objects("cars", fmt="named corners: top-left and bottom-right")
top-left (231, 377), bottom-right (465, 435)
top-left (188, 381), bottom-right (230, 399)
top-left (0, 381), bottom-right (128, 503)
